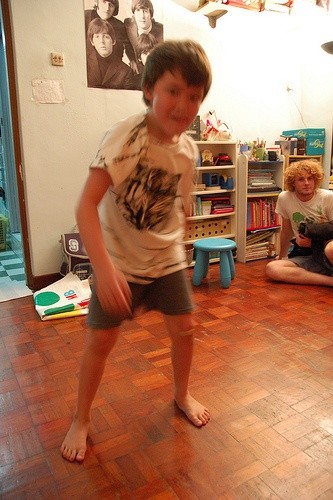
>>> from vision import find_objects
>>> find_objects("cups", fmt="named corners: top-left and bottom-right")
top-left (256, 148), bottom-right (266, 160)
top-left (268, 151), bottom-right (279, 161)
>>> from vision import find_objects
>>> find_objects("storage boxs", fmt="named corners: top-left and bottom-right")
top-left (0, 215), bottom-right (9, 252)
top-left (282, 129), bottom-right (325, 155)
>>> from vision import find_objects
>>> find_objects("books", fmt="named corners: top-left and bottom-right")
top-left (248, 168), bottom-right (281, 192)
top-left (246, 231), bottom-right (278, 260)
top-left (247, 199), bottom-right (281, 228)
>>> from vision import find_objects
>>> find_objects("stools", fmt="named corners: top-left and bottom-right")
top-left (192, 238), bottom-right (236, 288)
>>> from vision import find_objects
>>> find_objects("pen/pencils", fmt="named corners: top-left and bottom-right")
top-left (256, 137), bottom-right (267, 147)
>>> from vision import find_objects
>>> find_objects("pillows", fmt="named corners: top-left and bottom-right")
top-left (289, 223), bottom-right (333, 258)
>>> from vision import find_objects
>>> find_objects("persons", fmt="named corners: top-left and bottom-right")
top-left (86, 17), bottom-right (134, 90)
top-left (84, 0), bottom-right (140, 75)
top-left (265, 159), bottom-right (333, 287)
top-left (124, 32), bottom-right (153, 90)
top-left (124, 0), bottom-right (163, 71)
top-left (59, 40), bottom-right (213, 462)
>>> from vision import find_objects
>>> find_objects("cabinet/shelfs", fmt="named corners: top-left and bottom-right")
top-left (184, 141), bottom-right (237, 268)
top-left (237, 155), bottom-right (284, 263)
top-left (286, 155), bottom-right (323, 169)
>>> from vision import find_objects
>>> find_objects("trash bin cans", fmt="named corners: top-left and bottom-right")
top-left (1, 216), bottom-right (9, 250)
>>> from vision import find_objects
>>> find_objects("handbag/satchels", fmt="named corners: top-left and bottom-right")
top-left (59, 225), bottom-right (94, 282)
top-left (288, 221), bottom-right (332, 259)
